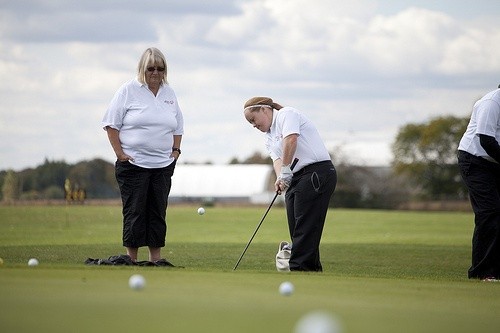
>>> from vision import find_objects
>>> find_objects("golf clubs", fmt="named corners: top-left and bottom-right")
top-left (232, 157), bottom-right (300, 271)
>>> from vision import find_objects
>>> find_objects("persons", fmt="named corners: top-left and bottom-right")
top-left (103, 47), bottom-right (183, 265)
top-left (457, 83), bottom-right (500, 281)
top-left (244, 97), bottom-right (338, 272)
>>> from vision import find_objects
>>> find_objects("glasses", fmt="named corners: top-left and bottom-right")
top-left (147, 66), bottom-right (165, 72)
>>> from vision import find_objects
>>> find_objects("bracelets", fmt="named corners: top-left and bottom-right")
top-left (173, 147), bottom-right (182, 154)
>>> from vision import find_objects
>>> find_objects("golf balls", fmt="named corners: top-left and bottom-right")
top-left (278, 281), bottom-right (295, 297)
top-left (28, 258), bottom-right (39, 267)
top-left (128, 274), bottom-right (147, 292)
top-left (198, 207), bottom-right (205, 215)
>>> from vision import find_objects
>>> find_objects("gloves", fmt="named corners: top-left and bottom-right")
top-left (280, 166), bottom-right (293, 183)
